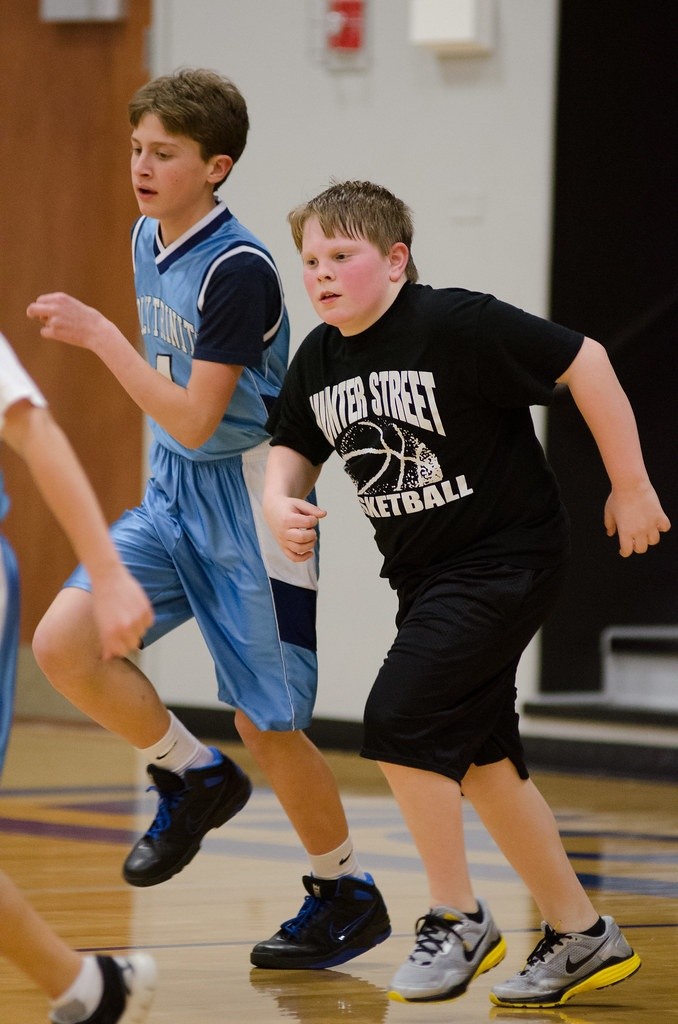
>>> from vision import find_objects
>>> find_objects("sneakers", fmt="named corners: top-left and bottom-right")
top-left (122, 746), bottom-right (251, 886)
top-left (51, 952), bottom-right (156, 1024)
top-left (490, 916), bottom-right (641, 1008)
top-left (388, 898), bottom-right (508, 1006)
top-left (250, 873), bottom-right (390, 969)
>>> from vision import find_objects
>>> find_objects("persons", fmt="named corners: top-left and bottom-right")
top-left (0, 334), bottom-right (157, 1024)
top-left (31, 69), bottom-right (394, 967)
top-left (263, 180), bottom-right (670, 1009)
